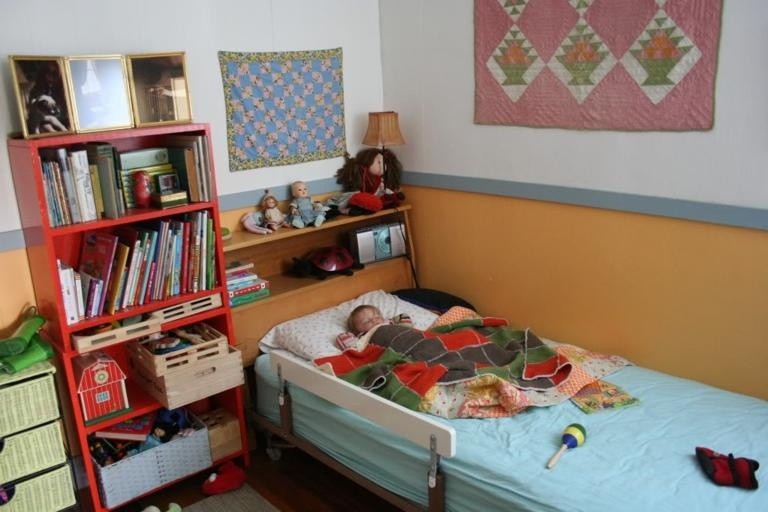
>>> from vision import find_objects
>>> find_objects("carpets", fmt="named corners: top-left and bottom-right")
top-left (181, 484), bottom-right (279, 512)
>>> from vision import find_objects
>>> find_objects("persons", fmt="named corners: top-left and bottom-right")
top-left (23, 63), bottom-right (66, 133)
top-left (336, 306), bottom-right (412, 355)
top-left (260, 190), bottom-right (288, 230)
top-left (341, 147), bottom-right (406, 207)
top-left (287, 181), bottom-right (327, 229)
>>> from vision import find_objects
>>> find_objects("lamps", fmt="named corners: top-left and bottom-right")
top-left (362, 111), bottom-right (407, 210)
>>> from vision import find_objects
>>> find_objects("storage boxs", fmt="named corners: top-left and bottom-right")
top-left (0, 361), bottom-right (82, 512)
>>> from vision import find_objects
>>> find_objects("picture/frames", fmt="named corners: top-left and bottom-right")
top-left (62, 54), bottom-right (135, 134)
top-left (126, 50), bottom-right (193, 129)
top-left (8, 54), bottom-right (76, 140)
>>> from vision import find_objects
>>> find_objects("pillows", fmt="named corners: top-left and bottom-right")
top-left (258, 288), bottom-right (439, 361)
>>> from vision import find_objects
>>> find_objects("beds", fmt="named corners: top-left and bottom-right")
top-left (246, 288), bottom-right (767, 511)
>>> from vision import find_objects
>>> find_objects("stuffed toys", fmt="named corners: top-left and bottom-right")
top-left (241, 210), bottom-right (272, 234)
top-left (288, 244), bottom-right (358, 283)
top-left (322, 200), bottom-right (369, 219)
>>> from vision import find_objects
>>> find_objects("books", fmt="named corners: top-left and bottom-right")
top-left (94, 413), bottom-right (158, 442)
top-left (226, 259), bottom-right (271, 308)
top-left (35, 133), bottom-right (221, 324)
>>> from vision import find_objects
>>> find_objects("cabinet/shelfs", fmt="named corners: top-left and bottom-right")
top-left (6, 122), bottom-right (251, 512)
top-left (221, 203), bottom-right (413, 370)
